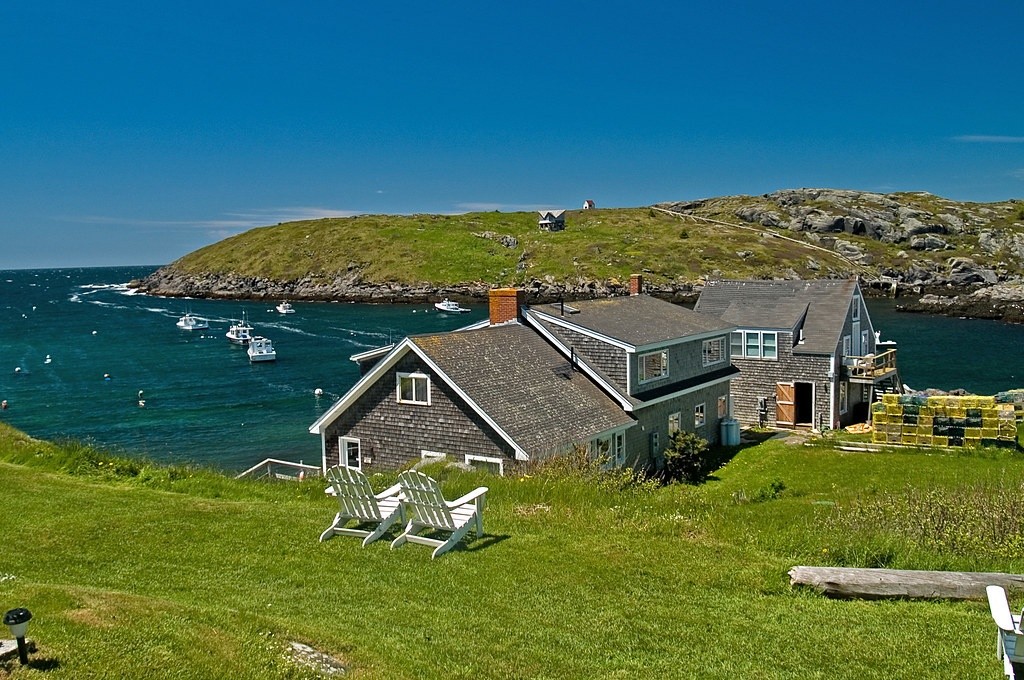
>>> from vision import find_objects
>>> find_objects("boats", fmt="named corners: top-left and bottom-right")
top-left (176, 309), bottom-right (209, 331)
top-left (276, 300), bottom-right (296, 313)
top-left (247, 336), bottom-right (277, 363)
top-left (435, 298), bottom-right (471, 315)
top-left (225, 311), bottom-right (254, 346)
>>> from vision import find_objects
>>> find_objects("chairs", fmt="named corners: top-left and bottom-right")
top-left (319, 464), bottom-right (411, 547)
top-left (389, 470), bottom-right (488, 561)
top-left (986, 586), bottom-right (1024, 680)
top-left (857, 353), bottom-right (874, 374)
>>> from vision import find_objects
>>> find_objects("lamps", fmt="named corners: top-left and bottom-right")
top-left (2, 608), bottom-right (33, 667)
